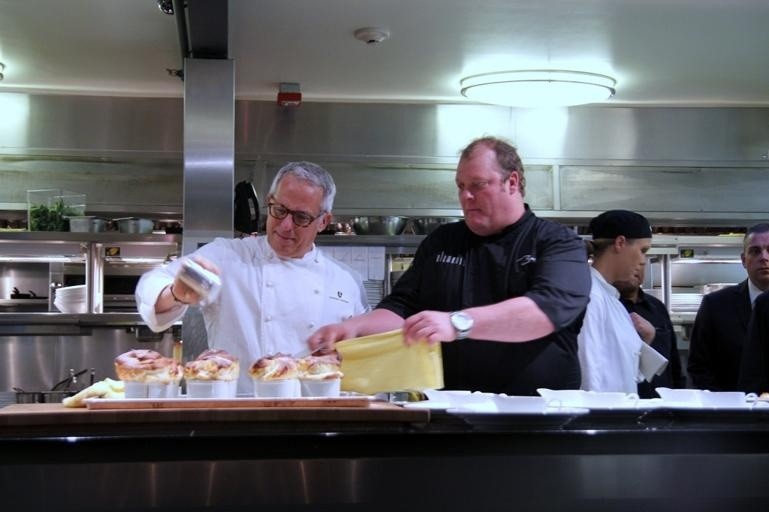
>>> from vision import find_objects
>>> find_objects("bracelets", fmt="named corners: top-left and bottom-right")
top-left (170, 286), bottom-right (189, 305)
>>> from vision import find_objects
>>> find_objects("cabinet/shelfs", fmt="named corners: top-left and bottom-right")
top-left (0, 229), bottom-right (183, 330)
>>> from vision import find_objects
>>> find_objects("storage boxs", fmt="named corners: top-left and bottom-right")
top-left (25, 187), bottom-right (88, 233)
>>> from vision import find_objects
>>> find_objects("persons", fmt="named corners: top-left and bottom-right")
top-left (578, 210), bottom-right (656, 397)
top-left (737, 291), bottom-right (769, 392)
top-left (614, 266), bottom-right (681, 400)
top-left (134, 160), bottom-right (370, 391)
top-left (686, 220), bottom-right (769, 389)
top-left (307, 136), bottom-right (592, 396)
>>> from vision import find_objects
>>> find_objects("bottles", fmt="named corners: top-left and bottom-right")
top-left (178, 259), bottom-right (223, 306)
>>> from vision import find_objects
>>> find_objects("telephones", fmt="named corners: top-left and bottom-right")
top-left (233, 180), bottom-right (261, 235)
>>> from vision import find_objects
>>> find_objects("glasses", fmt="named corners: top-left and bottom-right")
top-left (268, 203), bottom-right (323, 227)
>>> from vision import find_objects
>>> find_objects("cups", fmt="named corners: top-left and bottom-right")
top-left (186, 379), bottom-right (237, 400)
top-left (120, 378), bottom-right (180, 399)
top-left (253, 380), bottom-right (301, 397)
top-left (301, 379), bottom-right (341, 397)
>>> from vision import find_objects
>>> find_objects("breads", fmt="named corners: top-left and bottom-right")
top-left (184, 348), bottom-right (239, 382)
top-left (247, 352), bottom-right (297, 382)
top-left (295, 346), bottom-right (345, 381)
top-left (113, 349), bottom-right (183, 384)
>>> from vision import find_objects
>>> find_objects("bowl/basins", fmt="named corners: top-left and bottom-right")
top-left (68, 218), bottom-right (105, 233)
top-left (350, 215), bottom-right (410, 236)
top-left (118, 218), bottom-right (154, 234)
top-left (54, 284), bottom-right (87, 315)
top-left (408, 217), bottom-right (460, 236)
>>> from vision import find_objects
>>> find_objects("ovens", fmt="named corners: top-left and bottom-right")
top-left (47, 259), bottom-right (162, 313)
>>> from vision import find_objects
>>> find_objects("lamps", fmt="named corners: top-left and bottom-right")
top-left (454, 55), bottom-right (621, 112)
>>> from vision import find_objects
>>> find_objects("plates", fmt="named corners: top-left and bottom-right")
top-left (417, 400), bottom-right (769, 434)
top-left (670, 291), bottom-right (706, 311)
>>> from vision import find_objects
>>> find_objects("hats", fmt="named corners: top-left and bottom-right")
top-left (590, 210), bottom-right (653, 239)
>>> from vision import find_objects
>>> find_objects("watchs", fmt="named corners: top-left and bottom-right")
top-left (449, 308), bottom-right (473, 344)
